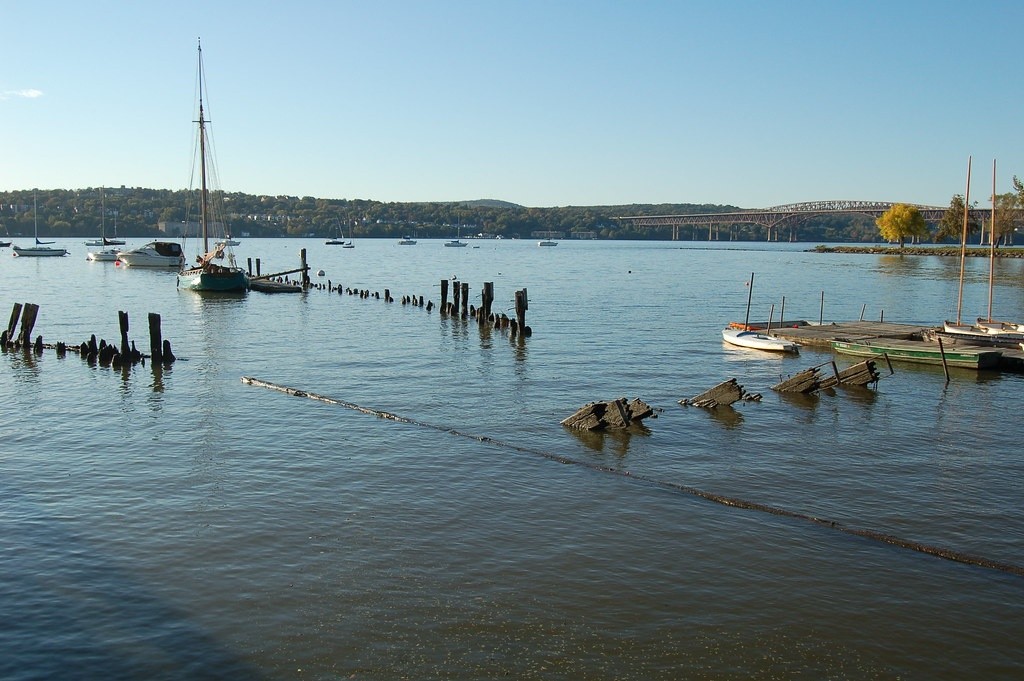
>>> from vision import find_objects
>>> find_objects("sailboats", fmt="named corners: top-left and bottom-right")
top-left (443, 212), bottom-right (469, 247)
top-left (178, 37), bottom-right (252, 298)
top-left (12, 188), bottom-right (71, 256)
top-left (84, 185), bottom-right (126, 245)
top-left (942, 154), bottom-right (1024, 341)
top-left (0, 209), bottom-right (16, 247)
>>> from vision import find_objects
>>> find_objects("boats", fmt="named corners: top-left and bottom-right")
top-left (87, 247), bottom-right (124, 261)
top-left (722, 324), bottom-right (798, 352)
top-left (398, 240), bottom-right (416, 245)
top-left (325, 238), bottom-right (355, 248)
top-left (116, 242), bottom-right (189, 269)
top-left (539, 240), bottom-right (558, 246)
top-left (215, 235), bottom-right (241, 246)
top-left (831, 339), bottom-right (1001, 371)
top-left (117, 241), bottom-right (186, 269)
